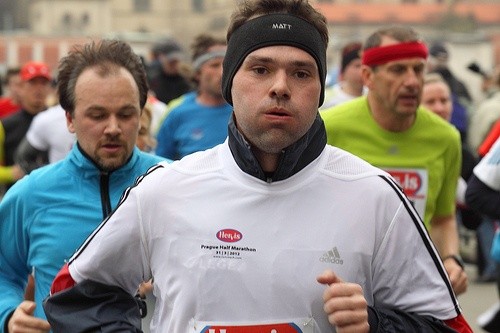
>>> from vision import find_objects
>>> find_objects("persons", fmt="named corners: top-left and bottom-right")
top-left (0, 53), bottom-right (167, 176)
top-left (149, 38), bottom-right (189, 106)
top-left (42, 0), bottom-right (475, 332)
top-left (153, 33), bottom-right (233, 162)
top-left (1, 38), bottom-right (173, 333)
top-left (319, 32), bottom-right (500, 333)
top-left (318, 26), bottom-right (469, 297)
top-left (0, 61), bottom-right (52, 195)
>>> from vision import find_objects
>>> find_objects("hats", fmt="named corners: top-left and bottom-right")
top-left (152, 38), bottom-right (184, 60)
top-left (20, 60), bottom-right (50, 81)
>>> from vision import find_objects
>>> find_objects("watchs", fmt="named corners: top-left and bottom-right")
top-left (443, 254), bottom-right (465, 271)
